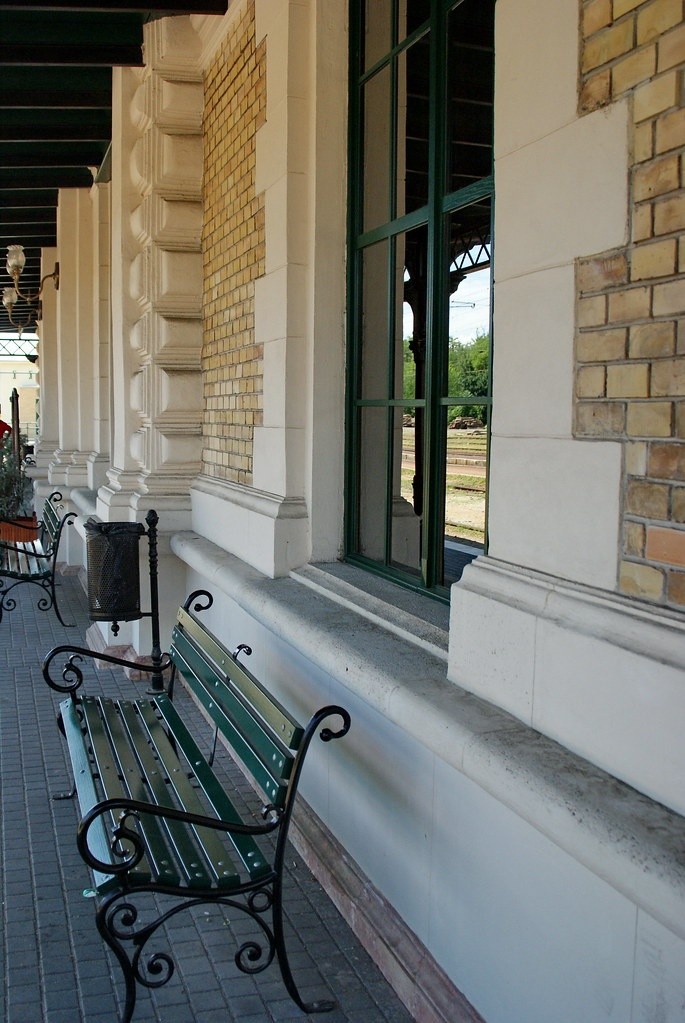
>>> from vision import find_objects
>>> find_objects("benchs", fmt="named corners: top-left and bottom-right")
top-left (43, 589), bottom-right (354, 1023)
top-left (0, 491), bottom-right (78, 627)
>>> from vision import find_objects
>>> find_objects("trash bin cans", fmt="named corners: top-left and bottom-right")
top-left (85, 521), bottom-right (143, 622)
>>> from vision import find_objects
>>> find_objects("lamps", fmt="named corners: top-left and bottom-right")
top-left (1, 288), bottom-right (42, 330)
top-left (6, 245), bottom-right (59, 300)
